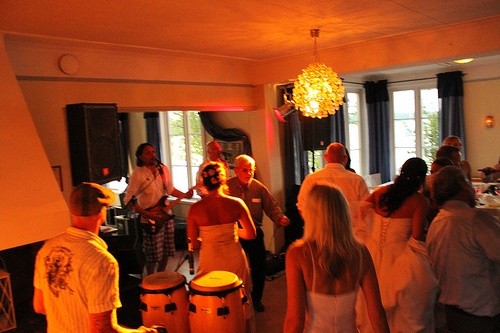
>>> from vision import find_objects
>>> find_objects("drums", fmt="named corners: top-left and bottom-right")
top-left (139, 272), bottom-right (189, 333)
top-left (189, 271), bottom-right (247, 333)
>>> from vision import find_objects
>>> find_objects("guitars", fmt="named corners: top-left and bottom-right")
top-left (137, 186), bottom-right (197, 235)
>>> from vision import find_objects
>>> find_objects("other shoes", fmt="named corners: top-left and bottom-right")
top-left (252, 303), bottom-right (264, 312)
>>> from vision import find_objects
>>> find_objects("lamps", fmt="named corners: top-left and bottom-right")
top-left (293, 28), bottom-right (346, 119)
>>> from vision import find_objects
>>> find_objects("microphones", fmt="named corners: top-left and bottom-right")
top-left (187, 238), bottom-right (194, 275)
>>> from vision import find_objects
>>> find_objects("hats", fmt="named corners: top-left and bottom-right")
top-left (69, 182), bottom-right (116, 216)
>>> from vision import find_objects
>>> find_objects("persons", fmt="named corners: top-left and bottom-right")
top-left (124, 143), bottom-right (194, 276)
top-left (422, 136), bottom-right (472, 240)
top-left (425, 164), bottom-right (500, 333)
top-left (283, 185), bottom-right (390, 333)
top-left (194, 142), bottom-right (230, 196)
top-left (226, 154), bottom-right (291, 312)
top-left (296, 142), bottom-right (370, 243)
top-left (33, 182), bottom-right (157, 333)
top-left (187, 162), bottom-right (256, 333)
top-left (359, 156), bottom-right (436, 333)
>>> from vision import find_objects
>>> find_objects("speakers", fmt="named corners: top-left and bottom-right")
top-left (303, 116), bottom-right (330, 150)
top-left (66, 102), bottom-right (123, 187)
